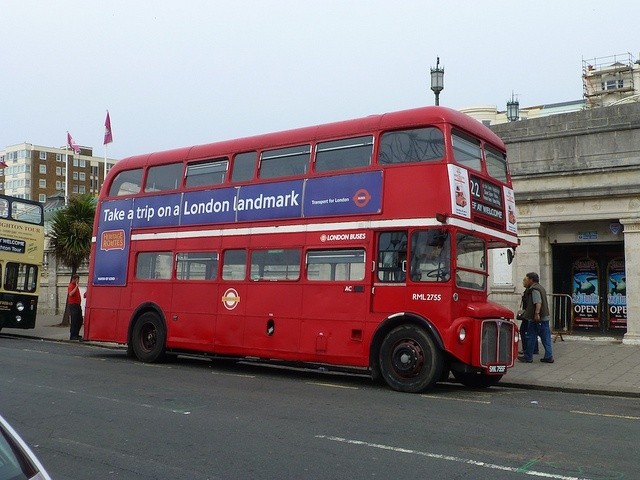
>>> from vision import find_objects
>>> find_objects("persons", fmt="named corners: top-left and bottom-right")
top-left (518, 272), bottom-right (554, 363)
top-left (68, 274), bottom-right (83, 340)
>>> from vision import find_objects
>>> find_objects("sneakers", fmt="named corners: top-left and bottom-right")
top-left (540, 358), bottom-right (555, 363)
top-left (518, 356), bottom-right (533, 363)
top-left (70, 335), bottom-right (82, 340)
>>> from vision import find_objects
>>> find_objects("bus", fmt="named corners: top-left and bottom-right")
top-left (0, 194), bottom-right (44, 334)
top-left (83, 106), bottom-right (521, 393)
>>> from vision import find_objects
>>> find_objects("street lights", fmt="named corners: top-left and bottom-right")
top-left (431, 58), bottom-right (444, 105)
top-left (506, 92), bottom-right (519, 122)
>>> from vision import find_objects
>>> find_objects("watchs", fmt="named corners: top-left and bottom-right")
top-left (535, 312), bottom-right (540, 315)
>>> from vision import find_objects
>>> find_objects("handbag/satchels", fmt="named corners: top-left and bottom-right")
top-left (517, 301), bottom-right (525, 320)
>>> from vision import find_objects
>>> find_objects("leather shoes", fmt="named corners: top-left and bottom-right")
top-left (518, 351), bottom-right (525, 355)
top-left (533, 352), bottom-right (538, 354)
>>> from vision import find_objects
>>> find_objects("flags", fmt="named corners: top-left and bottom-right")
top-left (67, 133), bottom-right (81, 153)
top-left (104, 112), bottom-right (112, 144)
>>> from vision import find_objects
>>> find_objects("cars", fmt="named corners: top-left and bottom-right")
top-left (1, 412), bottom-right (52, 480)
